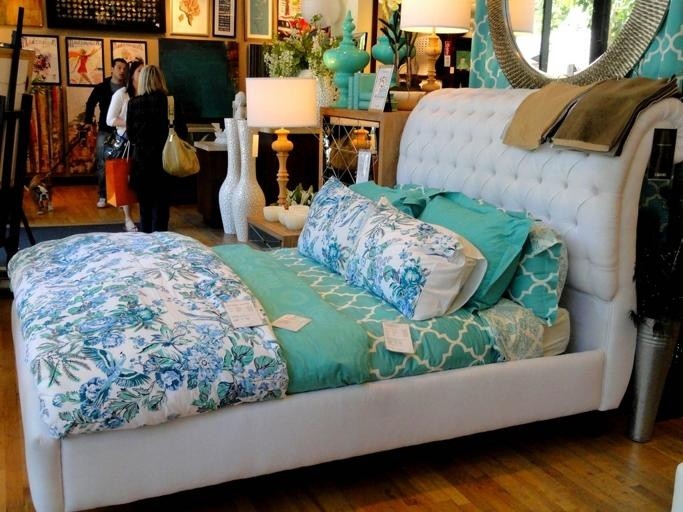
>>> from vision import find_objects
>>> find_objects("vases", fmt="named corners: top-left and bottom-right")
top-left (298, 70), bottom-right (330, 108)
top-left (218, 118), bottom-right (241, 236)
top-left (232, 121), bottom-right (266, 243)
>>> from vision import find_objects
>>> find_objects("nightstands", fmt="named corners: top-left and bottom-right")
top-left (247, 216), bottom-right (303, 250)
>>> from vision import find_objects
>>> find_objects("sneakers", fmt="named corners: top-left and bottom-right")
top-left (96, 198), bottom-right (107, 209)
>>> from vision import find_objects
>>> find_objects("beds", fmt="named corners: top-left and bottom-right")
top-left (8, 88), bottom-right (683, 512)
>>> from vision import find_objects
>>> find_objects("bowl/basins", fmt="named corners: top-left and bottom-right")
top-left (278, 209), bottom-right (292, 226)
top-left (289, 204), bottom-right (309, 214)
top-left (263, 205), bottom-right (283, 222)
top-left (287, 213), bottom-right (306, 230)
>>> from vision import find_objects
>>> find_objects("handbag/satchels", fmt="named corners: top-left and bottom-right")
top-left (162, 128), bottom-right (201, 177)
top-left (99, 131), bottom-right (129, 162)
top-left (104, 159), bottom-right (140, 207)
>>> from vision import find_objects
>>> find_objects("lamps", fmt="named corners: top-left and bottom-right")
top-left (371, 16), bottom-right (407, 88)
top-left (246, 77), bottom-right (320, 208)
top-left (401, 0), bottom-right (474, 90)
top-left (507, 0), bottom-right (534, 38)
top-left (323, 13), bottom-right (369, 109)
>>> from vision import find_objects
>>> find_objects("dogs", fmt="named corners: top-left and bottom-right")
top-left (22, 183), bottom-right (54, 218)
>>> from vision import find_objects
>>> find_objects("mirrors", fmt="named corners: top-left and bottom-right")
top-left (487, 0), bottom-right (671, 88)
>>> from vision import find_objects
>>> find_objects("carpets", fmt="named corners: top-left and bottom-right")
top-left (0, 223), bottom-right (143, 267)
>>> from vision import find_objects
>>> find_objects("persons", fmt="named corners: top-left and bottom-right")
top-left (74, 46), bottom-right (98, 84)
top-left (105, 61), bottom-right (143, 232)
top-left (126, 65), bottom-right (189, 232)
top-left (83, 58), bottom-right (127, 209)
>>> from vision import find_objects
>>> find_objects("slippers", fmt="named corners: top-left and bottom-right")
top-left (123, 223), bottom-right (139, 232)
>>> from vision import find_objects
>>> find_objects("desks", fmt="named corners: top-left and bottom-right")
top-left (192, 140), bottom-right (228, 228)
top-left (186, 122), bottom-right (218, 143)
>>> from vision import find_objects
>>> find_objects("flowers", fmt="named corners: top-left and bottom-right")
top-left (263, 15), bottom-right (339, 103)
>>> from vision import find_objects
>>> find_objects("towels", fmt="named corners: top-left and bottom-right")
top-left (501, 77), bottom-right (679, 156)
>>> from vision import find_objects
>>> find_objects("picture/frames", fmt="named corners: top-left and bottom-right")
top-left (0, 0), bottom-right (278, 86)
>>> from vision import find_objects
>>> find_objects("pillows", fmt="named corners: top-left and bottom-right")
top-left (298, 176), bottom-right (570, 326)
